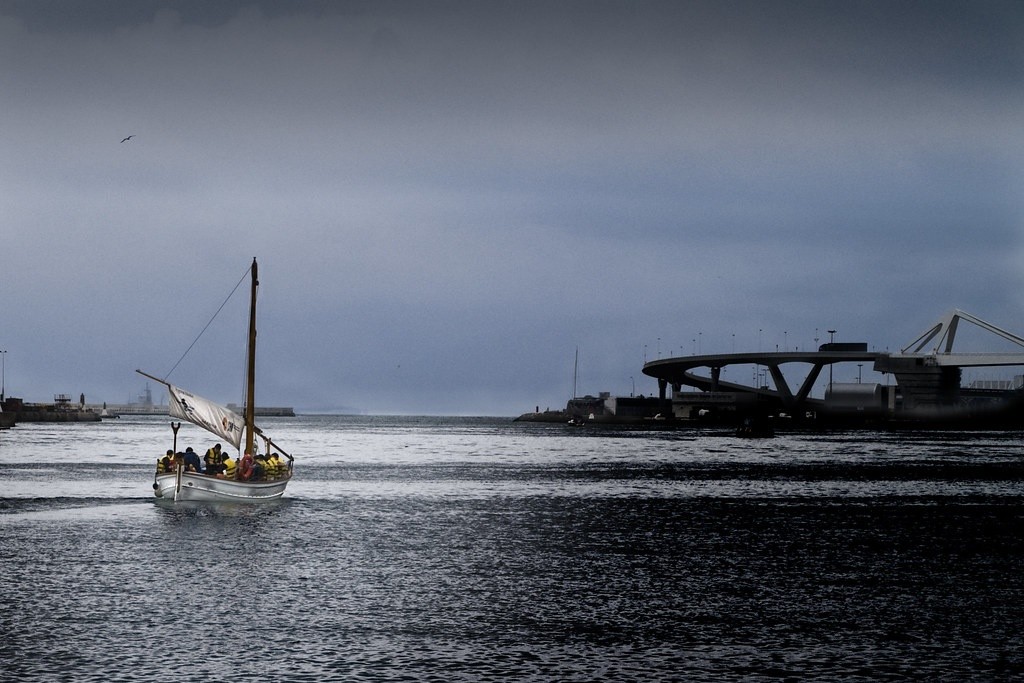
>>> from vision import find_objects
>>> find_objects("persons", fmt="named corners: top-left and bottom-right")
top-left (254, 453), bottom-right (288, 480)
top-left (203, 444), bottom-right (239, 479)
top-left (157, 447), bottom-right (202, 474)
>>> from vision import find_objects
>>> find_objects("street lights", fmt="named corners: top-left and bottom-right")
top-left (857, 365), bottom-right (862, 384)
top-left (828, 330), bottom-right (838, 400)
top-left (630, 328), bottom-right (820, 397)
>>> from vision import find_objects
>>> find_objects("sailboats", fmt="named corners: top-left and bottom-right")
top-left (568, 348), bottom-right (586, 428)
top-left (137, 256), bottom-right (295, 502)
